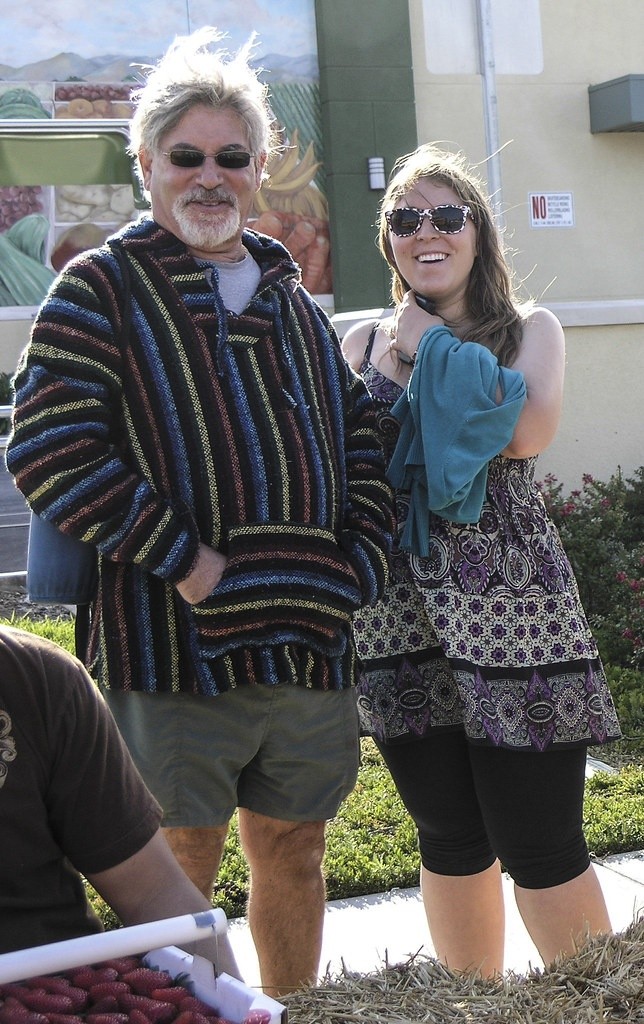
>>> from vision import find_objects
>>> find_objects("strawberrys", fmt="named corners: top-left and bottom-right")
top-left (0, 956), bottom-right (271, 1024)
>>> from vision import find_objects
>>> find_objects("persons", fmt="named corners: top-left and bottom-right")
top-left (341, 148), bottom-right (618, 981)
top-left (0, 620), bottom-right (243, 986)
top-left (4, 22), bottom-right (392, 1003)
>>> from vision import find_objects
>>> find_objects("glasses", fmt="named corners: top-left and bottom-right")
top-left (156, 149), bottom-right (258, 168)
top-left (384, 203), bottom-right (475, 234)
